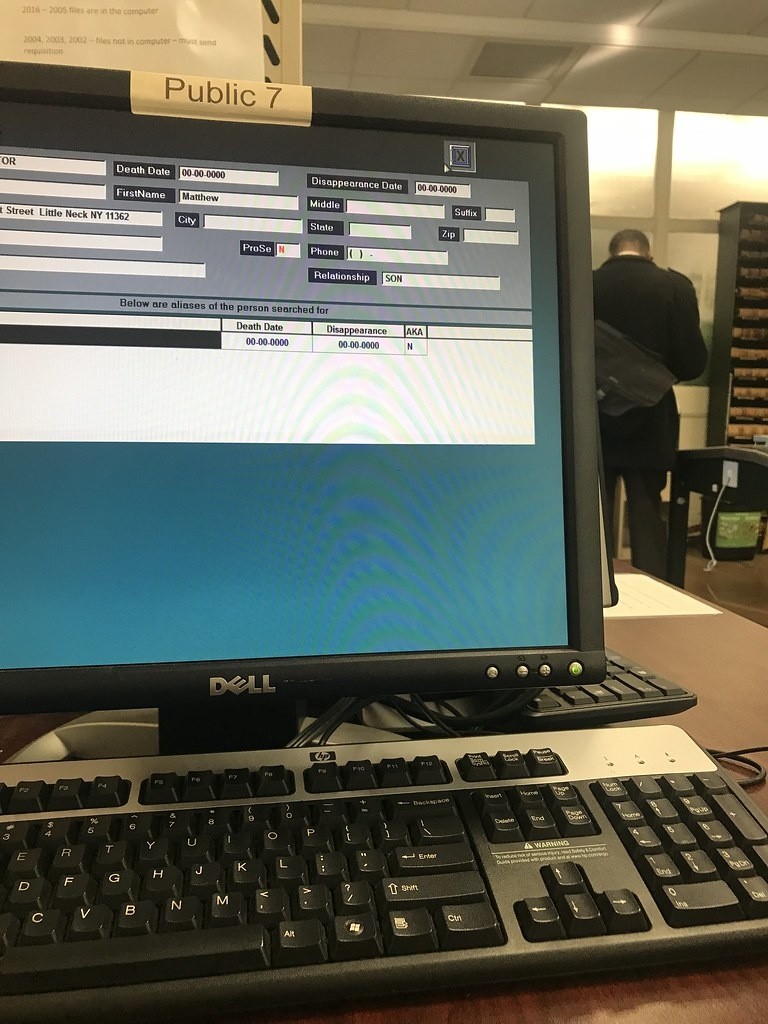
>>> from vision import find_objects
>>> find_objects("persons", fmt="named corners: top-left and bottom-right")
top-left (592, 230), bottom-right (710, 577)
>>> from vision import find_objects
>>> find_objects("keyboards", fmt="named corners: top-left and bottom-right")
top-left (493, 644), bottom-right (698, 730)
top-left (0, 722), bottom-right (768, 1024)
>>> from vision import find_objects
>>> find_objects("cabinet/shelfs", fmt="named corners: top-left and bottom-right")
top-left (706, 200), bottom-right (768, 446)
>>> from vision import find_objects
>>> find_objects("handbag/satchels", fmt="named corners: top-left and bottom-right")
top-left (594, 319), bottom-right (678, 416)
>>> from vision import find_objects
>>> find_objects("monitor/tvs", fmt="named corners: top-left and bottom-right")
top-left (1, 60), bottom-right (607, 755)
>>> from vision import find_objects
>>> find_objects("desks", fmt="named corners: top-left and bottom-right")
top-left (0, 556), bottom-right (768, 1024)
